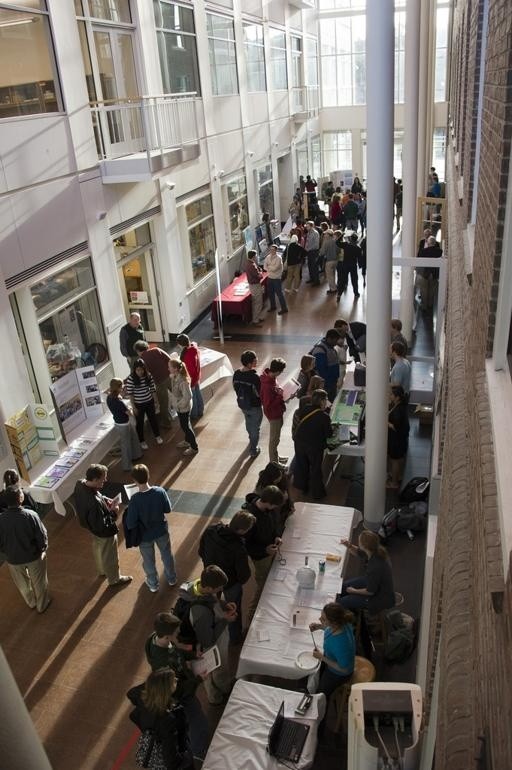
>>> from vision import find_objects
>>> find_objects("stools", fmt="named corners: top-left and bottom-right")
top-left (332, 655), bottom-right (376, 734)
top-left (354, 590), bottom-right (404, 664)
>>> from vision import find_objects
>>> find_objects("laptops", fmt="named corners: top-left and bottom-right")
top-left (267, 701), bottom-right (310, 763)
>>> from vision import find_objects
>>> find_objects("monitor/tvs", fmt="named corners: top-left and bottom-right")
top-left (357, 402), bottom-right (365, 446)
top-left (274, 237), bottom-right (281, 247)
top-left (259, 239), bottom-right (268, 252)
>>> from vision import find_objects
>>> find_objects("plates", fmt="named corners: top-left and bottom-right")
top-left (296, 649), bottom-right (320, 672)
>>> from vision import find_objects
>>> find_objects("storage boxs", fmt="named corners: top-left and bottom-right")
top-left (3, 404), bottom-right (68, 484)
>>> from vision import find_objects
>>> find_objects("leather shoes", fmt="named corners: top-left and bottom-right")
top-left (278, 310), bottom-right (288, 315)
top-left (113, 575), bottom-right (133, 585)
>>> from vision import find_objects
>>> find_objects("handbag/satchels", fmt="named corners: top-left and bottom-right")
top-left (135, 733), bottom-right (168, 770)
top-left (283, 260), bottom-right (288, 271)
top-left (379, 476), bottom-right (429, 538)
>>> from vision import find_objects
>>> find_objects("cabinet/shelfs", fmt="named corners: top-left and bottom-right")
top-left (0, 73), bottom-right (119, 154)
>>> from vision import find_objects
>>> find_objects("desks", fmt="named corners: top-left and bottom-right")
top-left (210, 230), bottom-right (293, 327)
top-left (320, 370), bottom-right (365, 488)
top-left (235, 501), bottom-right (364, 693)
top-left (28, 398), bottom-right (136, 521)
top-left (200, 678), bottom-right (324, 770)
top-left (180, 345), bottom-right (234, 397)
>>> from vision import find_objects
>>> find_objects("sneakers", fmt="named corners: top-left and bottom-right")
top-left (145, 575), bottom-right (159, 592)
top-left (155, 436), bottom-right (163, 445)
top-left (140, 441), bottom-right (149, 450)
top-left (182, 447), bottom-right (198, 456)
top-left (176, 440), bottom-right (190, 448)
top-left (278, 456), bottom-right (289, 462)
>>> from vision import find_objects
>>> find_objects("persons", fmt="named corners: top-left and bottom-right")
top-left (173, 463), bottom-right (296, 709)
top-left (330, 531), bottom-right (398, 609)
top-left (308, 602), bottom-right (356, 699)
top-left (133, 339), bottom-right (173, 430)
top-left (292, 321), bottom-right (410, 501)
top-left (1, 468), bottom-right (39, 513)
top-left (75, 311), bottom-right (101, 348)
top-left (260, 359), bottom-right (298, 471)
top-left (127, 665), bottom-right (195, 770)
top-left (107, 377), bottom-right (144, 475)
top-left (232, 350), bottom-right (262, 458)
top-left (122, 462), bottom-right (178, 592)
top-left (0, 487), bottom-right (52, 613)
top-left (72, 464), bottom-right (134, 586)
top-left (120, 311), bottom-right (146, 372)
top-left (126, 357), bottom-right (163, 450)
top-left (247, 166), bottom-right (445, 315)
top-left (144, 612), bottom-right (208, 706)
top-left (170, 334), bottom-right (204, 456)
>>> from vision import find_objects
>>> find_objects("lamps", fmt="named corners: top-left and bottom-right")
top-left (0, 16), bottom-right (41, 28)
top-left (165, 181), bottom-right (176, 190)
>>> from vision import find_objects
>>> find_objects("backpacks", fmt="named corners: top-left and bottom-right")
top-left (171, 598), bottom-right (216, 652)
top-left (384, 610), bottom-right (420, 665)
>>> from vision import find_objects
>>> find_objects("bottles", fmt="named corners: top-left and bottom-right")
top-left (294, 555), bottom-right (317, 589)
